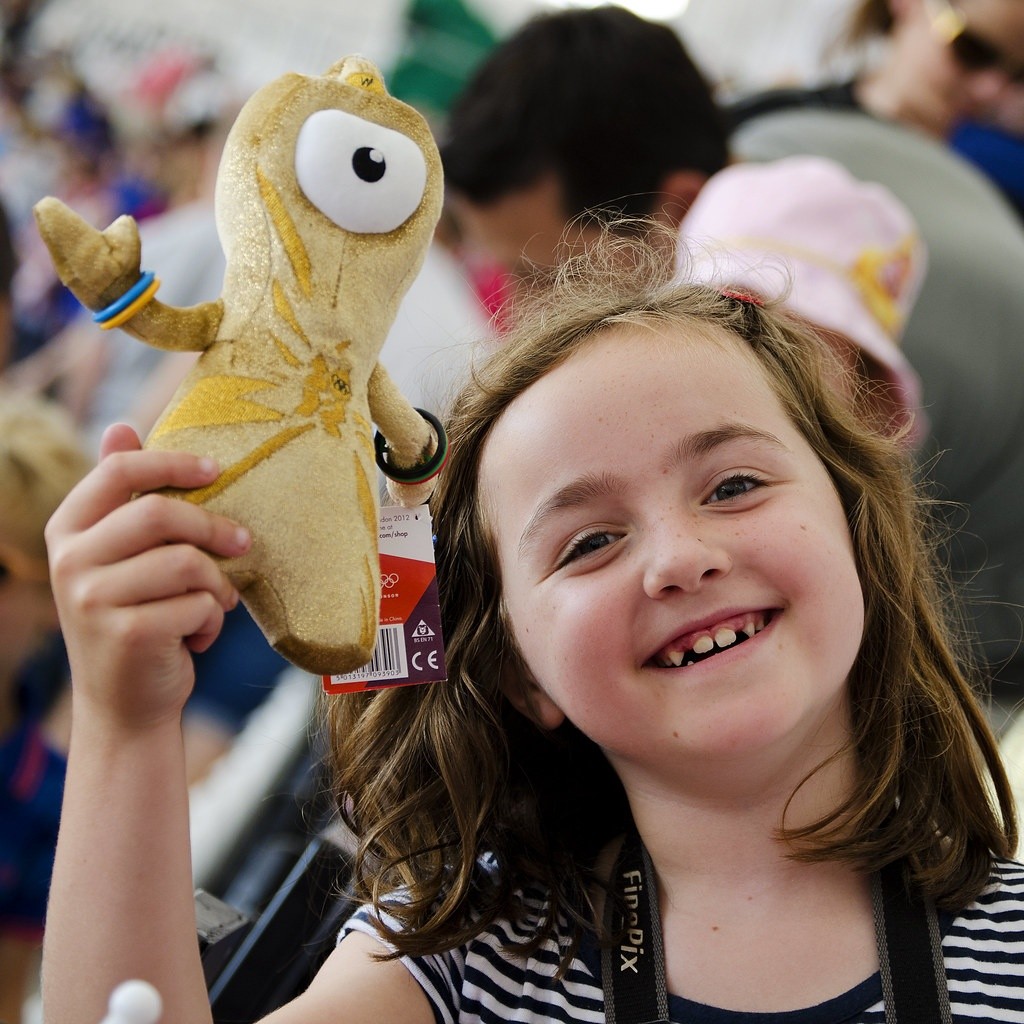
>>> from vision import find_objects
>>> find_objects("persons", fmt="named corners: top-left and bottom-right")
top-left (38, 288), bottom-right (1024, 1024)
top-left (0, 0), bottom-right (1024, 933)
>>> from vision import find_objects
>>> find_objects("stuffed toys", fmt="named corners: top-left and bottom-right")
top-left (36, 57), bottom-right (450, 693)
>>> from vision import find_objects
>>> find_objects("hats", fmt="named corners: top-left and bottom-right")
top-left (656, 156), bottom-right (930, 455)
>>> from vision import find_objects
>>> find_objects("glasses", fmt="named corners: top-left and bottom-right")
top-left (923, 0), bottom-right (1024, 90)
